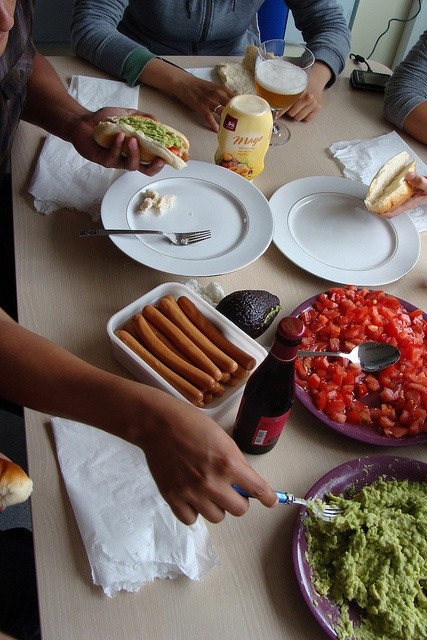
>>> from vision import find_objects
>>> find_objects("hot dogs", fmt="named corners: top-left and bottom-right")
top-left (91, 114), bottom-right (190, 170)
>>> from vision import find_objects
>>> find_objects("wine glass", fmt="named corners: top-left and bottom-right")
top-left (254, 38), bottom-right (316, 146)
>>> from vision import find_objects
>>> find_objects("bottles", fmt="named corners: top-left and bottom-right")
top-left (232, 316), bottom-right (306, 457)
top-left (213, 94), bottom-right (273, 184)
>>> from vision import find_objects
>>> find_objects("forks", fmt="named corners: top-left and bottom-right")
top-left (79, 228), bottom-right (211, 246)
top-left (231, 484), bottom-right (344, 523)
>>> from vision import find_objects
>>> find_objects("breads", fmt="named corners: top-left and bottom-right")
top-left (217, 45), bottom-right (275, 95)
top-left (0, 457), bottom-right (33, 510)
top-left (365, 150), bottom-right (416, 213)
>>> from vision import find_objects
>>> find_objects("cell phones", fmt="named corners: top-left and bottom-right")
top-left (348, 70), bottom-right (394, 96)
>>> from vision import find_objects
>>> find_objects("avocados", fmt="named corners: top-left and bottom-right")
top-left (217, 289), bottom-right (280, 339)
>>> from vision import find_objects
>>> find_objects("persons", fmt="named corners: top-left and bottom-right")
top-left (383, 30), bottom-right (427, 146)
top-left (1, 0), bottom-right (280, 526)
top-left (71, 0), bottom-right (352, 133)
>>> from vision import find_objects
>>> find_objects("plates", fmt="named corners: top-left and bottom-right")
top-left (292, 456), bottom-right (427, 640)
top-left (268, 175), bottom-right (420, 286)
top-left (100, 160), bottom-right (274, 278)
top-left (288, 288), bottom-right (427, 448)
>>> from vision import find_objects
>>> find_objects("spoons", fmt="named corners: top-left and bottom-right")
top-left (261, 342), bottom-right (401, 372)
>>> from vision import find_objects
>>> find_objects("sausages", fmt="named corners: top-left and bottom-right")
top-left (117, 295), bottom-right (256, 407)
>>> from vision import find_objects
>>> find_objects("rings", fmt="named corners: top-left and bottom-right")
top-left (214, 105), bottom-right (222, 112)
